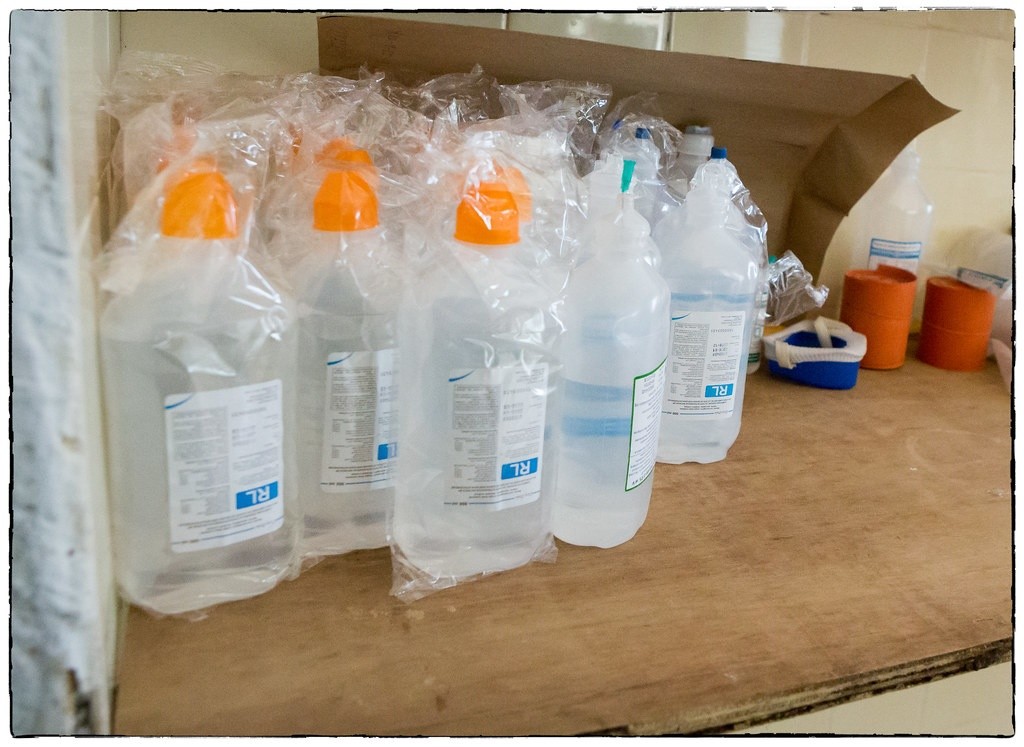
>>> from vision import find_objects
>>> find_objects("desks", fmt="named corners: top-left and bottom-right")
top-left (104, 330), bottom-right (1014, 736)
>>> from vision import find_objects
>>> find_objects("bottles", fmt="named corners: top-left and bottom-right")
top-left (854, 152), bottom-right (932, 333)
top-left (100, 97), bottom-right (768, 614)
